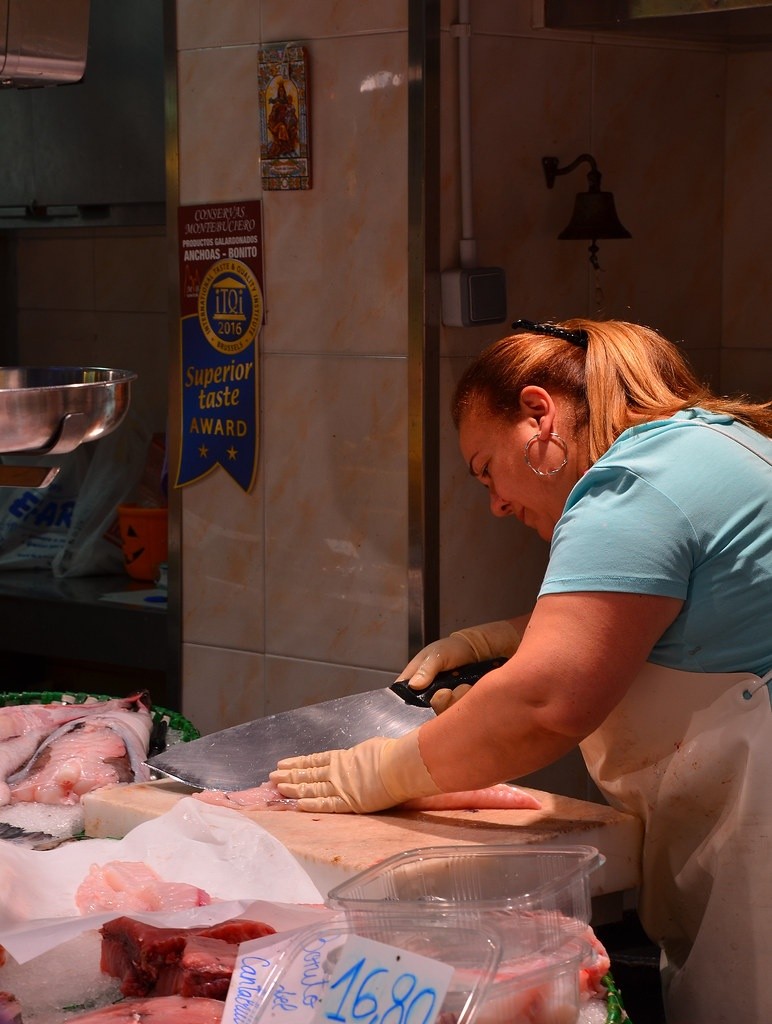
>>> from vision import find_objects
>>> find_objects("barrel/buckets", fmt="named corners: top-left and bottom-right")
top-left (115, 501), bottom-right (169, 583)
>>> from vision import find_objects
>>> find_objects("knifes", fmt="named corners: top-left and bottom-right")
top-left (142, 656), bottom-right (510, 792)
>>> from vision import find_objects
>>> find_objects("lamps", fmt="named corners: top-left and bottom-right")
top-left (542, 152), bottom-right (633, 306)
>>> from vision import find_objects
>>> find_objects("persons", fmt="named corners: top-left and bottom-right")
top-left (269, 322), bottom-right (772, 1024)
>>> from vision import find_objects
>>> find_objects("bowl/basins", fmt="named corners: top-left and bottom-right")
top-left (324, 844), bottom-right (604, 1024)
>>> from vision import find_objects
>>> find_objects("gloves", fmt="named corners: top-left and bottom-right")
top-left (269, 724), bottom-right (445, 813)
top-left (395, 619), bottom-right (521, 714)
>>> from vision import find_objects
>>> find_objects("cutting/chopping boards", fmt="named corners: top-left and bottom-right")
top-left (79, 769), bottom-right (645, 911)
